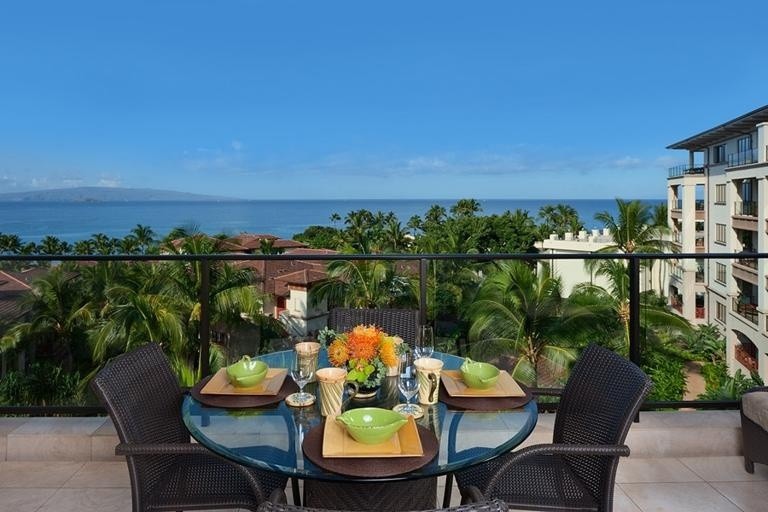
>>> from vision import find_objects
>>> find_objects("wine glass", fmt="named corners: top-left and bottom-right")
top-left (290, 349), bottom-right (315, 402)
top-left (398, 361), bottom-right (420, 413)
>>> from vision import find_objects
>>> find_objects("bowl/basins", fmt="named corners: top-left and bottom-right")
top-left (460, 355), bottom-right (501, 389)
top-left (225, 354), bottom-right (268, 387)
top-left (336, 406), bottom-right (409, 445)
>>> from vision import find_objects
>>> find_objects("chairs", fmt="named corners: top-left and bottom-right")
top-left (442, 343), bottom-right (651, 511)
top-left (301, 306), bottom-right (438, 512)
top-left (88, 338), bottom-right (300, 511)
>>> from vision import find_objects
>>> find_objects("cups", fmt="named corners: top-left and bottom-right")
top-left (413, 358), bottom-right (444, 405)
top-left (414, 323), bottom-right (435, 358)
top-left (295, 342), bottom-right (320, 383)
top-left (316, 366), bottom-right (359, 417)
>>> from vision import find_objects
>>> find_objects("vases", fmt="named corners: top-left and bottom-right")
top-left (343, 373), bottom-right (381, 403)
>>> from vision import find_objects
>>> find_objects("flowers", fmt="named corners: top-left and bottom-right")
top-left (317, 321), bottom-right (408, 388)
